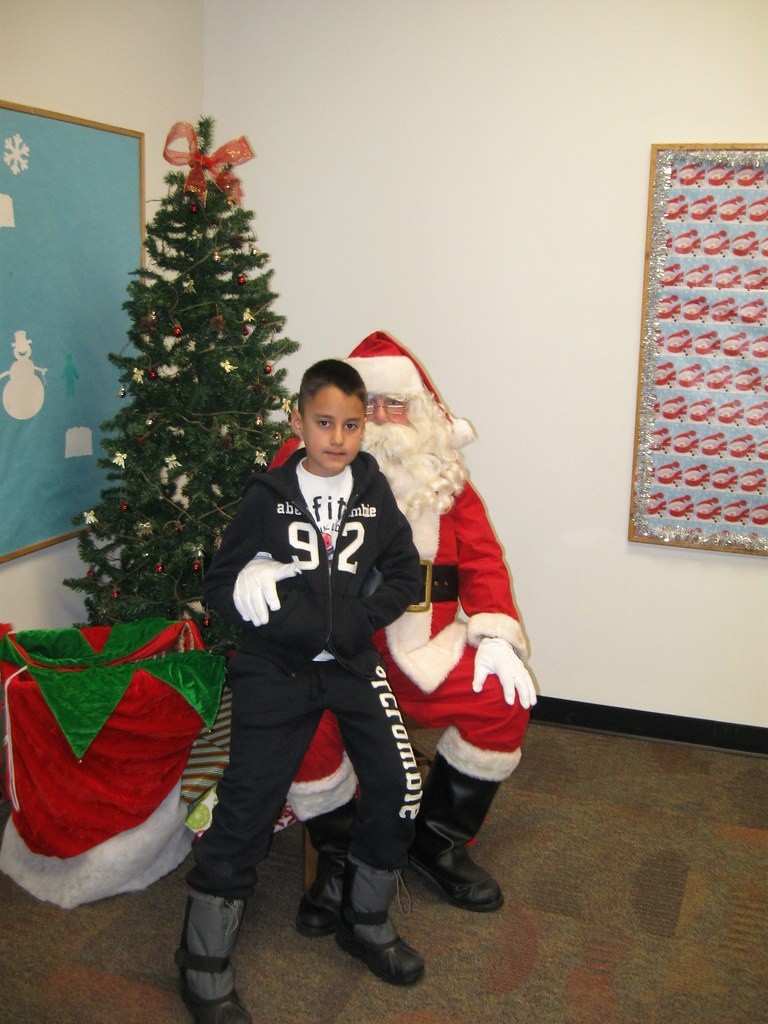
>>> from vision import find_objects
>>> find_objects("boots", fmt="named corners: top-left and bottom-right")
top-left (288, 750), bottom-right (359, 935)
top-left (173, 886), bottom-right (256, 1024)
top-left (335, 852), bottom-right (425, 984)
top-left (405, 724), bottom-right (521, 912)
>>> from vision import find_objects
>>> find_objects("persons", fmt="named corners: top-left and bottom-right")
top-left (269, 328), bottom-right (537, 934)
top-left (178, 358), bottom-right (423, 1023)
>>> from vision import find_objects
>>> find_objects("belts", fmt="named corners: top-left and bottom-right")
top-left (402, 560), bottom-right (459, 612)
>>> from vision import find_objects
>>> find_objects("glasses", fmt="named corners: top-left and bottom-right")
top-left (366, 403), bottom-right (407, 415)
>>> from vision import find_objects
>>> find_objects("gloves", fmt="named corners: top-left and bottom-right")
top-left (232, 551), bottom-right (298, 627)
top-left (472, 637), bottom-right (538, 710)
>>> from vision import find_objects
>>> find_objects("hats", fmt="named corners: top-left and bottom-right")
top-left (343, 331), bottom-right (473, 448)
